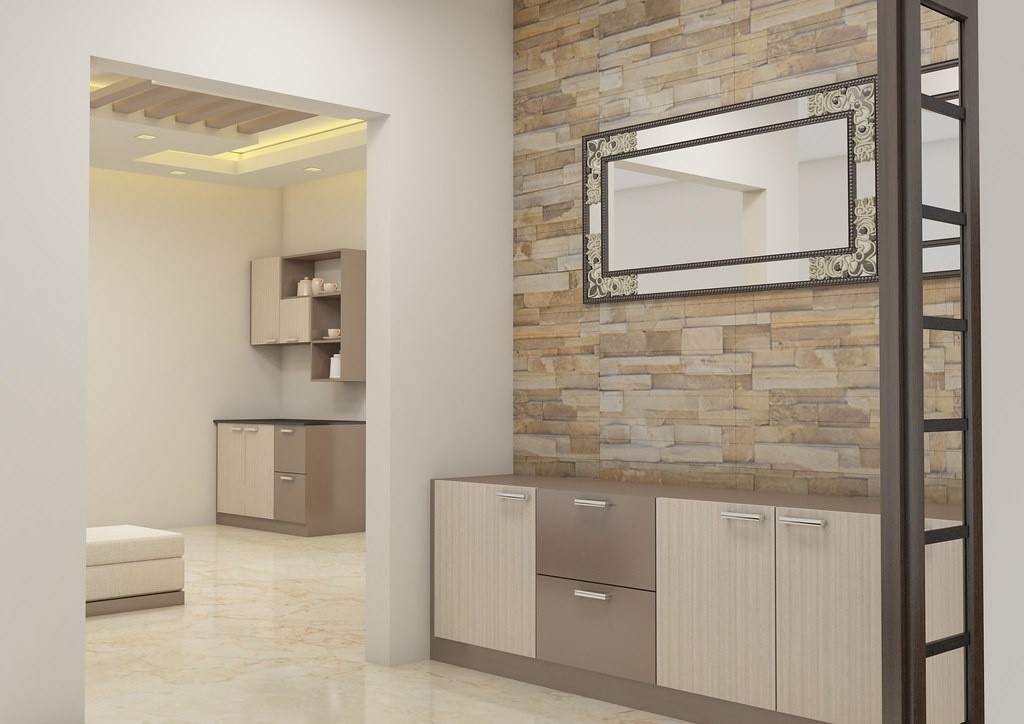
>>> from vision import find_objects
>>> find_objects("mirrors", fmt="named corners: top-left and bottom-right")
top-left (581, 74), bottom-right (878, 310)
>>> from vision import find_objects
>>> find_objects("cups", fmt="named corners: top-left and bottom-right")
top-left (311, 278), bottom-right (323, 295)
top-left (324, 283), bottom-right (337, 291)
top-left (328, 328), bottom-right (341, 336)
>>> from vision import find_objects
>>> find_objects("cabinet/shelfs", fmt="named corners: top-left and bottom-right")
top-left (656, 488), bottom-right (966, 724)
top-left (530, 478), bottom-right (658, 712)
top-left (424, 473), bottom-right (537, 689)
top-left (251, 245), bottom-right (365, 382)
top-left (212, 423), bottom-right (369, 538)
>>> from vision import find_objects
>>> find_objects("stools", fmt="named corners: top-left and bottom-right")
top-left (86, 523), bottom-right (185, 620)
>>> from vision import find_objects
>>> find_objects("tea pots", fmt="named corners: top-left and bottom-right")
top-left (296, 277), bottom-right (310, 296)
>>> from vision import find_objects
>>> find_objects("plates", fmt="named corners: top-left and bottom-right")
top-left (322, 336), bottom-right (341, 339)
top-left (320, 290), bottom-right (342, 294)
